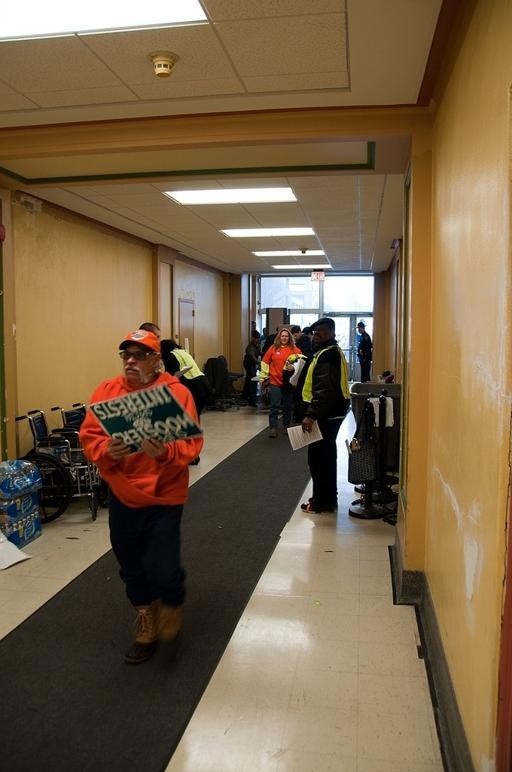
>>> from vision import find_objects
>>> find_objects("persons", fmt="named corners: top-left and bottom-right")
top-left (242, 320), bottom-right (315, 439)
top-left (139, 322), bottom-right (183, 380)
top-left (297, 316), bottom-right (349, 513)
top-left (77, 329), bottom-right (207, 664)
top-left (357, 322), bottom-right (375, 382)
top-left (160, 338), bottom-right (209, 465)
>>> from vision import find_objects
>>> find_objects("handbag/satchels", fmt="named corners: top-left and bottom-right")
top-left (346, 400), bottom-right (374, 485)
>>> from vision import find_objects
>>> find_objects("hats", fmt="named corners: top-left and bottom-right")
top-left (118, 329), bottom-right (160, 352)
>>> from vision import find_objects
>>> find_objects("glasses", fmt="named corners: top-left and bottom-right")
top-left (116, 350), bottom-right (151, 360)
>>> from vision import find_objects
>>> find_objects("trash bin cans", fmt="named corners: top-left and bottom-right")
top-left (348, 383), bottom-right (400, 474)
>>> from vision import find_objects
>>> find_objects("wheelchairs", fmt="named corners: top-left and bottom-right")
top-left (14, 409), bottom-right (102, 524)
top-left (51, 402), bottom-right (110, 508)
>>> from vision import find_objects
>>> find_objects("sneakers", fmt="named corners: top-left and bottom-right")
top-left (123, 603), bottom-right (157, 662)
top-left (268, 427), bottom-right (277, 437)
top-left (301, 497), bottom-right (337, 514)
top-left (158, 608), bottom-right (183, 640)
